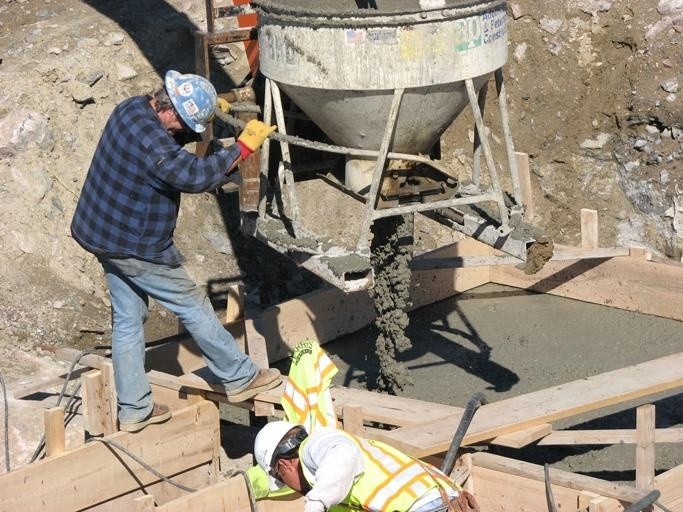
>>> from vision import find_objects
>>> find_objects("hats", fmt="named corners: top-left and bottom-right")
top-left (165, 69), bottom-right (217, 134)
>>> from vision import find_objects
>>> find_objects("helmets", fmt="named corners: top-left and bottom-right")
top-left (253, 420), bottom-right (306, 492)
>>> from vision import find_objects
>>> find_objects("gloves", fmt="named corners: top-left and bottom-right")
top-left (236, 119), bottom-right (276, 161)
top-left (215, 97), bottom-right (230, 114)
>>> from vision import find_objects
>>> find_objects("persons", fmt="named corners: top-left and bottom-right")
top-left (253, 420), bottom-right (480, 512)
top-left (69, 70), bottom-right (284, 433)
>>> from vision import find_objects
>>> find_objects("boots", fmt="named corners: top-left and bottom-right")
top-left (119, 403), bottom-right (172, 432)
top-left (227, 368), bottom-right (282, 403)
